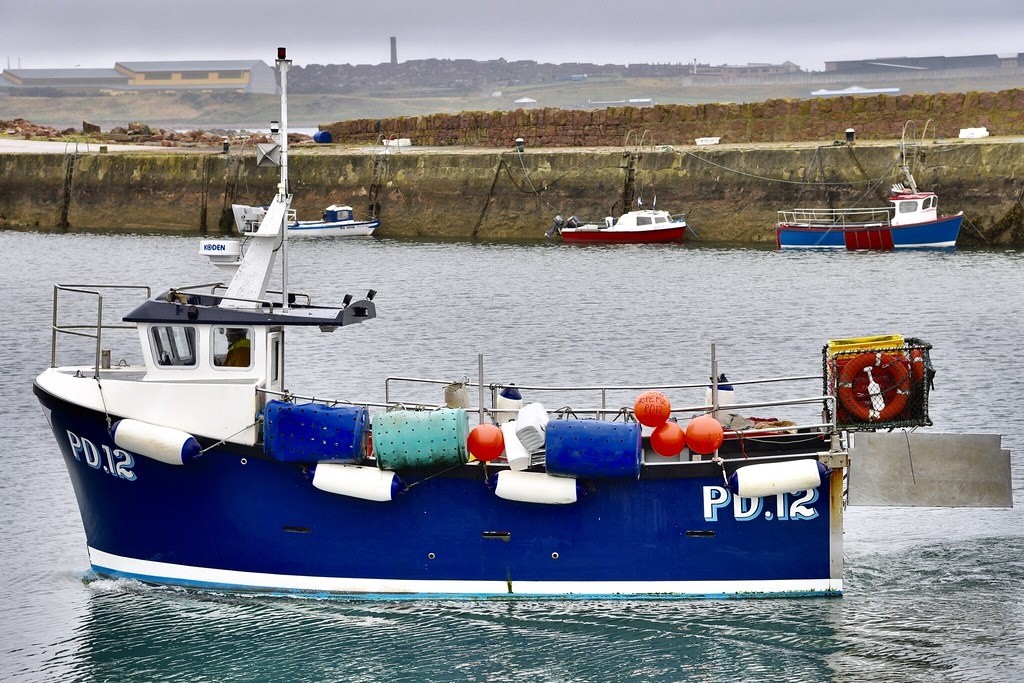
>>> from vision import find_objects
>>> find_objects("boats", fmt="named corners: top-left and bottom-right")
top-left (381, 138), bottom-right (412, 147)
top-left (693, 136), bottom-right (722, 146)
top-left (771, 139), bottom-right (965, 252)
top-left (30, 48), bottom-right (940, 612)
top-left (810, 86), bottom-right (901, 99)
top-left (543, 152), bottom-right (697, 246)
top-left (958, 126), bottom-right (989, 139)
top-left (228, 201), bottom-right (381, 238)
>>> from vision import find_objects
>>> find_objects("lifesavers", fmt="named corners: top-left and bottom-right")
top-left (908, 345), bottom-right (928, 385)
top-left (839, 352), bottom-right (910, 425)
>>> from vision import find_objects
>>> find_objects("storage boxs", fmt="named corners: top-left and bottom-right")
top-left (827, 333), bottom-right (912, 423)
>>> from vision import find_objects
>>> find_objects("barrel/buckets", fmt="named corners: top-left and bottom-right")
top-left (502, 419), bottom-right (529, 470)
top-left (516, 402), bottom-right (548, 452)
top-left (313, 131), bottom-right (332, 143)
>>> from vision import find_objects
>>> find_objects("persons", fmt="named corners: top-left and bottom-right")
top-left (223, 328), bottom-right (250, 367)
